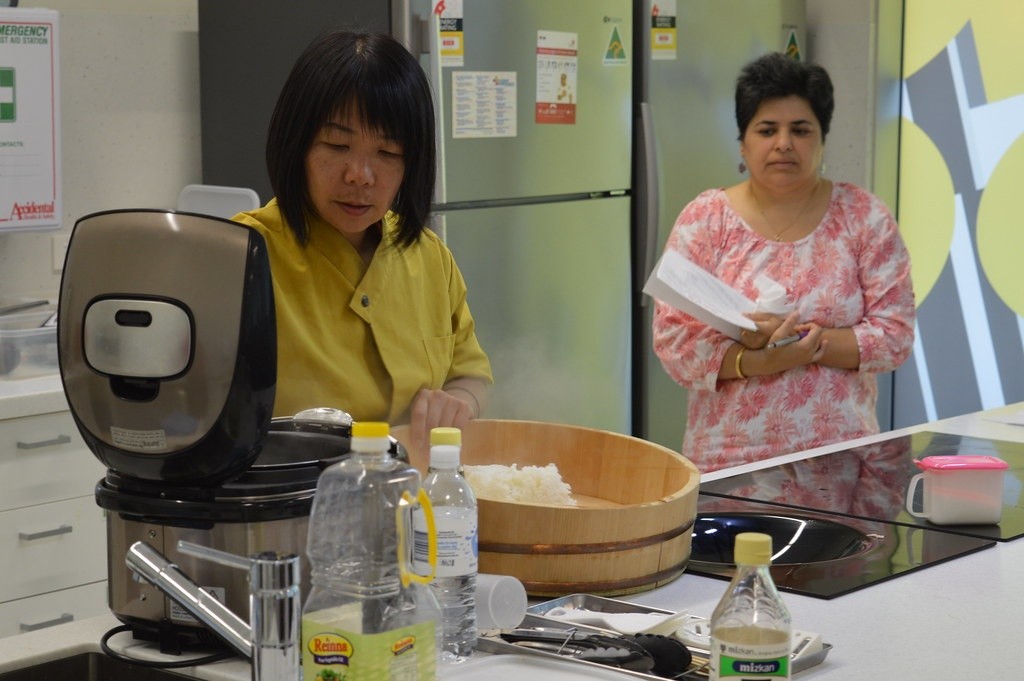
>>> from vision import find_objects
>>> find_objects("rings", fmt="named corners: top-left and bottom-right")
top-left (741, 331), bottom-right (746, 335)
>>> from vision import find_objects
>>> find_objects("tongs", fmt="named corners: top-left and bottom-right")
top-left (451, 627), bottom-right (693, 678)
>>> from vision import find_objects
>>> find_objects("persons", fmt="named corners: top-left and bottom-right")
top-left (230, 31), bottom-right (493, 449)
top-left (700, 436), bottom-right (914, 586)
top-left (651, 53), bottom-right (915, 472)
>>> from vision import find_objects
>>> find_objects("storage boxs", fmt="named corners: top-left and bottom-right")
top-left (0, 312), bottom-right (60, 381)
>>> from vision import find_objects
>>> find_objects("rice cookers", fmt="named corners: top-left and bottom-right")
top-left (56, 199), bottom-right (410, 666)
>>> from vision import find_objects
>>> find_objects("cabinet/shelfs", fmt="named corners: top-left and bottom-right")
top-left (0, 371), bottom-right (114, 637)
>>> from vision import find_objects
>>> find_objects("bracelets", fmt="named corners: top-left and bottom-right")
top-left (735, 347), bottom-right (746, 379)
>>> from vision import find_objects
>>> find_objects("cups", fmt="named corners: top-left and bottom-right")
top-left (473, 572), bottom-right (528, 635)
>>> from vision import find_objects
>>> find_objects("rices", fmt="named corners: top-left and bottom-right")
top-left (461, 458), bottom-right (577, 507)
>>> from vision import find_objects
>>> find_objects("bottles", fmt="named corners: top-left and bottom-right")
top-left (300, 422), bottom-right (442, 681)
top-left (708, 531), bottom-right (793, 681)
top-left (423, 426), bottom-right (473, 511)
top-left (409, 446), bottom-right (480, 666)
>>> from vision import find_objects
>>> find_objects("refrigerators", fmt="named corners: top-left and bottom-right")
top-left (192, 0), bottom-right (637, 476)
top-left (638, 0), bottom-right (814, 474)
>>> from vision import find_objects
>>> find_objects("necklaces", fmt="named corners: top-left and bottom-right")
top-left (750, 181), bottom-right (819, 242)
